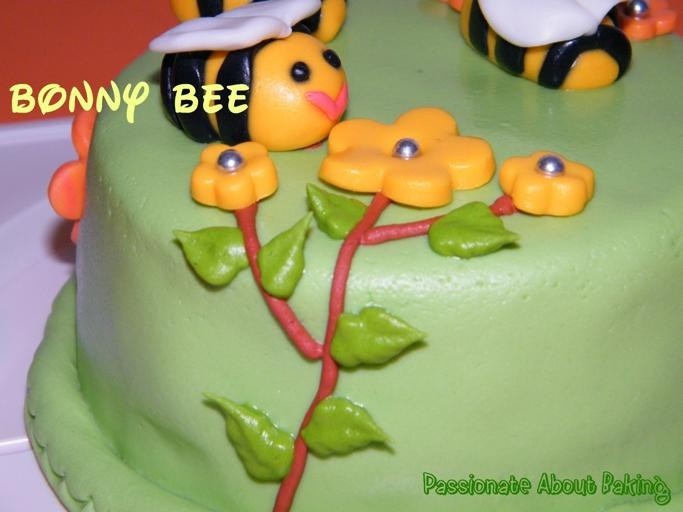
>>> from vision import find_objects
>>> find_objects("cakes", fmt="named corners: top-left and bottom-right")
top-left (23, 0), bottom-right (683, 512)
